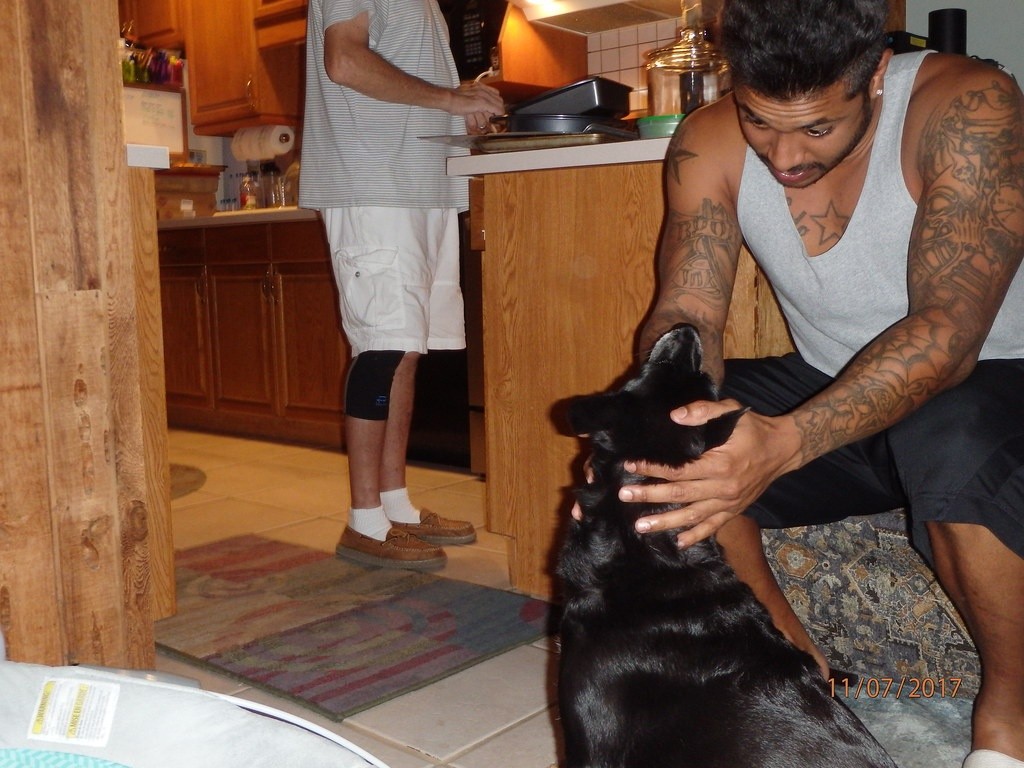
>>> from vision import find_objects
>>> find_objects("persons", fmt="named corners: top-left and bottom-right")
top-left (298, 1), bottom-right (505, 570)
top-left (570, 1), bottom-right (1024, 767)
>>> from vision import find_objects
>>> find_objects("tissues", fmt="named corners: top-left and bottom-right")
top-left (122, 87), bottom-right (183, 170)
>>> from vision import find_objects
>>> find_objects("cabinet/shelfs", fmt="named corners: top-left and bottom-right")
top-left (157, 208), bottom-right (349, 452)
top-left (119, 0), bottom-right (589, 138)
top-left (447, 135), bottom-right (793, 601)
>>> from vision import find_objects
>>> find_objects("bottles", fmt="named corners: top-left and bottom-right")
top-left (240, 173), bottom-right (261, 210)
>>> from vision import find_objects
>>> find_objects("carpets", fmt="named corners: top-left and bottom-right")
top-left (151, 533), bottom-right (549, 724)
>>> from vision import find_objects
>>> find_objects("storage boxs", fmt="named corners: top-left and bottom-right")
top-left (511, 76), bottom-right (634, 119)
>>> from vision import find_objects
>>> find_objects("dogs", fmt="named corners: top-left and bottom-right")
top-left (549, 321), bottom-right (899, 768)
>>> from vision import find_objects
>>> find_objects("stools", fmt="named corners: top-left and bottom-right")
top-left (758, 507), bottom-right (980, 699)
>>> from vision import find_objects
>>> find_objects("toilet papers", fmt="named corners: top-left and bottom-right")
top-left (231, 124), bottom-right (295, 161)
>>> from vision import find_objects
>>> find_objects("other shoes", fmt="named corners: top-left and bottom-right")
top-left (334, 524), bottom-right (447, 568)
top-left (390, 506), bottom-right (477, 543)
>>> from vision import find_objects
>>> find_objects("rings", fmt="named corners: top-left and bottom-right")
top-left (479, 125), bottom-right (487, 130)
